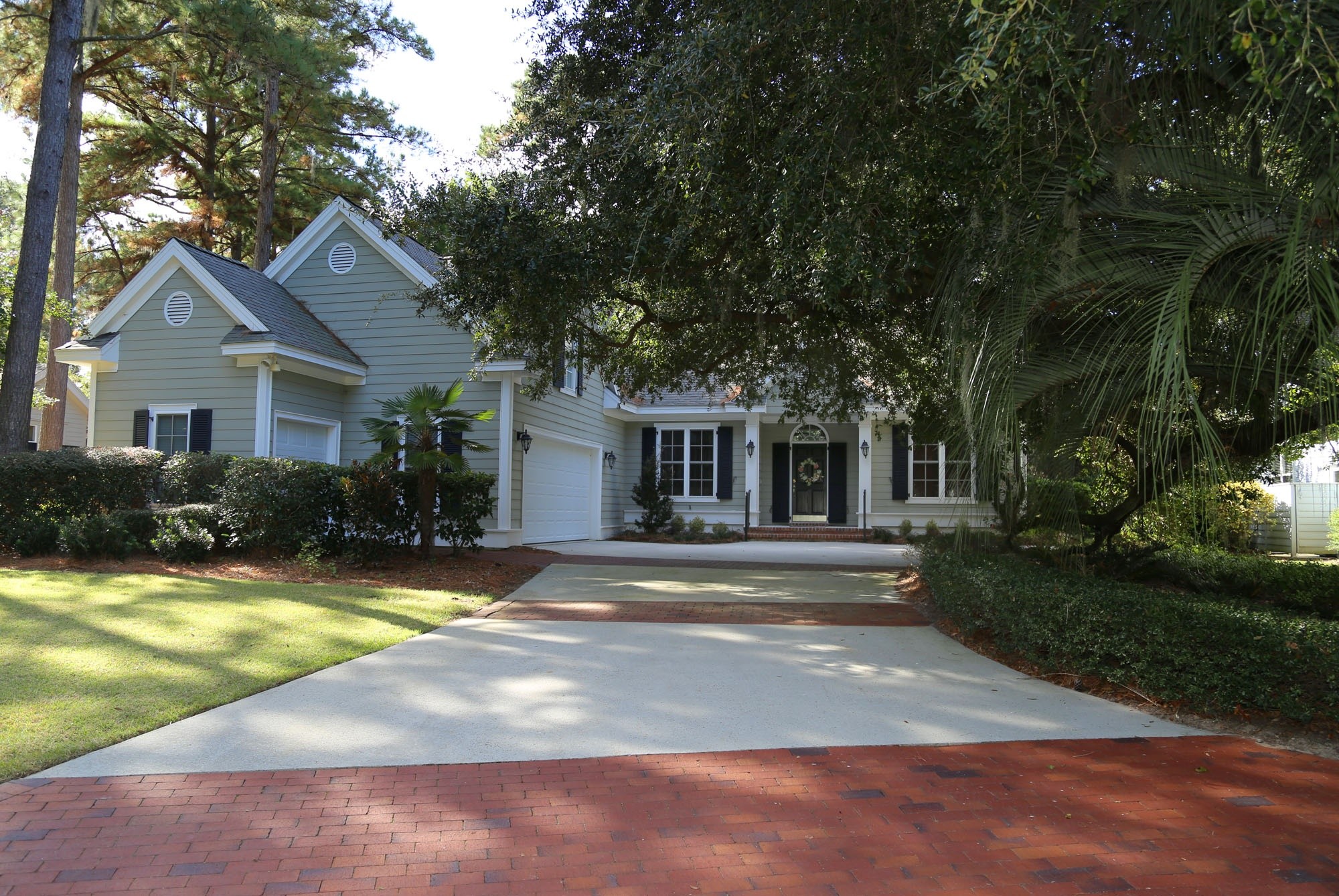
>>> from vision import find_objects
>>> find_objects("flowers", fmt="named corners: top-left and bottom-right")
top-left (798, 457), bottom-right (823, 487)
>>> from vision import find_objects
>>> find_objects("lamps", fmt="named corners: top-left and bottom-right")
top-left (860, 439), bottom-right (870, 459)
top-left (604, 450), bottom-right (616, 470)
top-left (745, 439), bottom-right (756, 458)
top-left (516, 428), bottom-right (533, 454)
